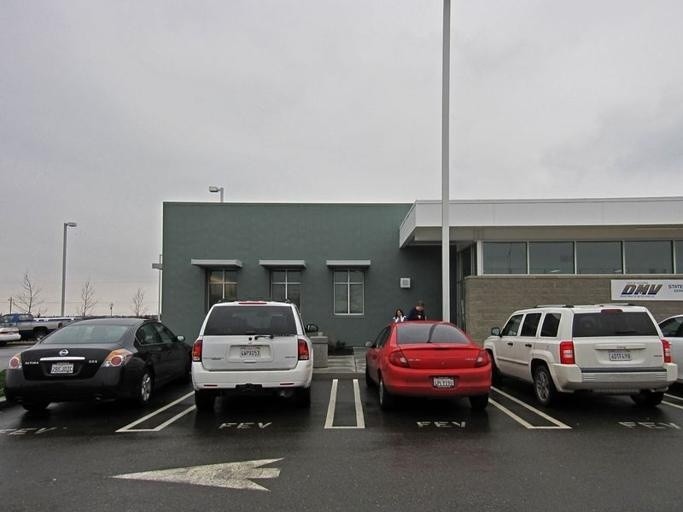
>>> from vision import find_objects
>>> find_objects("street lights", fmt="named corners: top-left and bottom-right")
top-left (60, 222), bottom-right (77, 317)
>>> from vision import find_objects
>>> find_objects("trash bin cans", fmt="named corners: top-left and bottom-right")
top-left (309, 336), bottom-right (328, 368)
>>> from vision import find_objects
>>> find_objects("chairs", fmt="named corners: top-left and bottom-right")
top-left (227, 313), bottom-right (288, 335)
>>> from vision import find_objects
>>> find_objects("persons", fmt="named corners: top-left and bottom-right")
top-left (408, 301), bottom-right (425, 321)
top-left (393, 309), bottom-right (406, 323)
